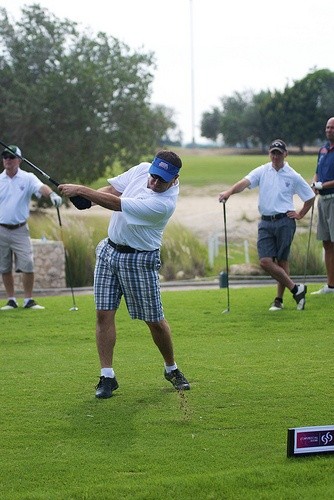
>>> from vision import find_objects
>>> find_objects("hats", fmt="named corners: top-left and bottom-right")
top-left (149, 157), bottom-right (179, 182)
top-left (269, 139), bottom-right (287, 153)
top-left (2, 145), bottom-right (22, 159)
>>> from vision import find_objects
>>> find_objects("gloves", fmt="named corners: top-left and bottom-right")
top-left (69, 196), bottom-right (92, 210)
top-left (49, 192), bottom-right (62, 207)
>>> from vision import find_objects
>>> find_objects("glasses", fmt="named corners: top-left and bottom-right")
top-left (4, 155), bottom-right (16, 159)
top-left (151, 174), bottom-right (167, 183)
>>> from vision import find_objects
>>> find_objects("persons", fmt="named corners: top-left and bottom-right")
top-left (310, 117), bottom-right (334, 296)
top-left (57, 152), bottom-right (190, 399)
top-left (0, 145), bottom-right (62, 311)
top-left (219, 140), bottom-right (316, 311)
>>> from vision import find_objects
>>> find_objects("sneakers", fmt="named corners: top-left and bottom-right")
top-left (95, 376), bottom-right (119, 399)
top-left (293, 284), bottom-right (307, 310)
top-left (269, 300), bottom-right (284, 311)
top-left (164, 369), bottom-right (190, 390)
top-left (311, 285), bottom-right (334, 294)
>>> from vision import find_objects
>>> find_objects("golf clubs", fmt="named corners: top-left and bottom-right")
top-left (54, 198), bottom-right (79, 312)
top-left (0, 142), bottom-right (91, 210)
top-left (221, 199), bottom-right (230, 314)
top-left (302, 193), bottom-right (315, 285)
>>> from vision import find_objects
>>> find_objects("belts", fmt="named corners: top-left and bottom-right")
top-left (0, 223), bottom-right (25, 230)
top-left (108, 238), bottom-right (142, 253)
top-left (261, 213), bottom-right (286, 222)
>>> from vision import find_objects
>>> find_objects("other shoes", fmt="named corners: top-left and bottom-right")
top-left (23, 300), bottom-right (45, 309)
top-left (1, 300), bottom-right (18, 310)
